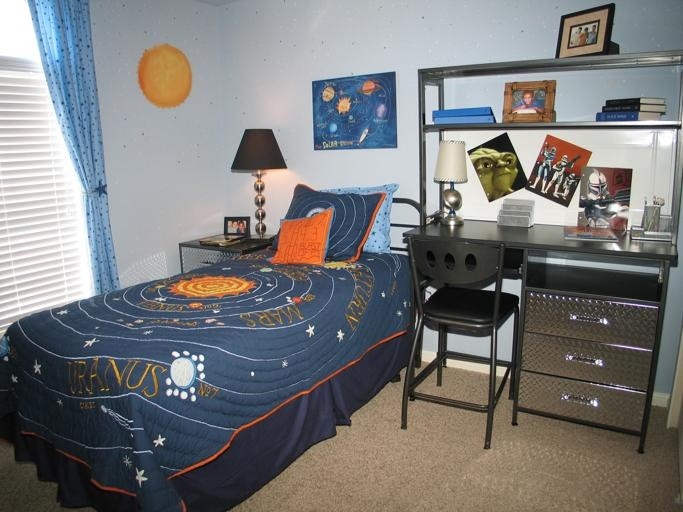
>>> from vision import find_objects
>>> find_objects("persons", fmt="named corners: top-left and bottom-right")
top-left (569, 24), bottom-right (597, 47)
top-left (236, 221), bottom-right (246, 234)
top-left (530, 147), bottom-right (576, 200)
top-left (580, 168), bottom-right (629, 229)
top-left (511, 89), bottom-right (544, 114)
top-left (228, 220), bottom-right (238, 234)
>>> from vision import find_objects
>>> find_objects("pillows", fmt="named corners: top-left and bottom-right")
top-left (272, 184), bottom-right (386, 264)
top-left (272, 206), bottom-right (335, 268)
top-left (316, 183), bottom-right (399, 254)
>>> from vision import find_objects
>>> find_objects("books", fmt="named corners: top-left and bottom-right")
top-left (595, 96), bottom-right (667, 121)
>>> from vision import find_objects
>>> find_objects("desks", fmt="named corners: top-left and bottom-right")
top-left (403, 220), bottom-right (678, 454)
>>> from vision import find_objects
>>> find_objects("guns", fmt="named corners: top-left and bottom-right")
top-left (568, 156), bottom-right (580, 167)
top-left (557, 165), bottom-right (568, 168)
top-left (573, 176), bottom-right (581, 186)
top-left (542, 141), bottom-right (549, 155)
top-left (580, 188), bottom-right (630, 206)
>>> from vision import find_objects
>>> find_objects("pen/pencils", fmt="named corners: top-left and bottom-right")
top-left (644, 196), bottom-right (665, 230)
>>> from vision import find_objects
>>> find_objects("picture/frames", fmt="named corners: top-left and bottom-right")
top-left (555, 2), bottom-right (619, 59)
top-left (502, 80), bottom-right (556, 123)
top-left (224, 217), bottom-right (249, 235)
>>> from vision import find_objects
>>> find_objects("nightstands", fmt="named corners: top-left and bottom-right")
top-left (179, 235), bottom-right (276, 273)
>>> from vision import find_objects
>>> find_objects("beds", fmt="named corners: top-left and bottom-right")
top-left (0, 197), bottom-right (425, 512)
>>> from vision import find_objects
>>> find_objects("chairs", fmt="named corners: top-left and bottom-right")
top-left (401, 237), bottom-right (519, 449)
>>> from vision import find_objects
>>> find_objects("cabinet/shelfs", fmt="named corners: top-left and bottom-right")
top-left (418, 50), bottom-right (683, 247)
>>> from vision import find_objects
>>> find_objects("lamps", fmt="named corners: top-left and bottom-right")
top-left (434, 139), bottom-right (467, 227)
top-left (231, 128), bottom-right (288, 243)
